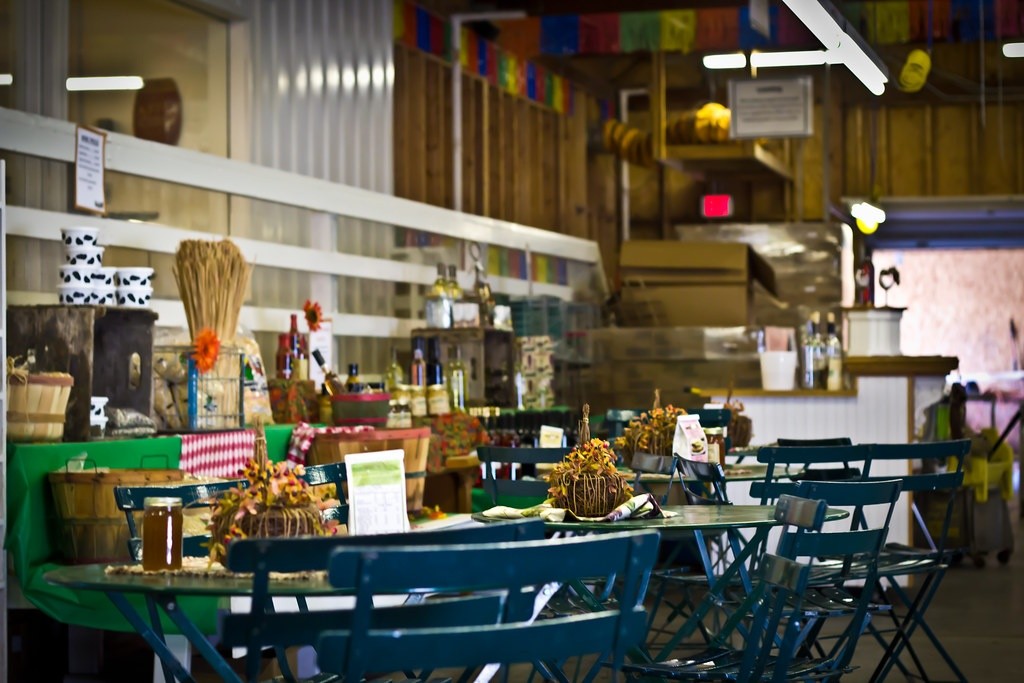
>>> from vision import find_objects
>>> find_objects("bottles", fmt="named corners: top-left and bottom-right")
top-left (142, 498), bottom-right (183, 570)
top-left (800, 319), bottom-right (841, 391)
top-left (276, 315), bottom-right (307, 379)
top-left (703, 428), bottom-right (725, 470)
top-left (433, 264), bottom-right (462, 303)
top-left (346, 363), bottom-right (362, 391)
top-left (311, 348), bottom-right (348, 394)
top-left (132, 76), bottom-right (183, 146)
top-left (410, 336), bottom-right (466, 415)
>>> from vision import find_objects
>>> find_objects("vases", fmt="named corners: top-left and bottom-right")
top-left (566, 477), bottom-right (618, 517)
top-left (240, 510), bottom-right (312, 537)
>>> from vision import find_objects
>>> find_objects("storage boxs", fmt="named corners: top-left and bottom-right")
top-left (411, 326), bottom-right (518, 408)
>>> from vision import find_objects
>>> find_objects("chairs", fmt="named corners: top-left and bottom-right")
top-left (115, 434), bottom-right (975, 683)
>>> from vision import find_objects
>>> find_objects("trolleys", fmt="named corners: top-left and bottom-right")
top-left (912, 382), bottom-right (1024, 570)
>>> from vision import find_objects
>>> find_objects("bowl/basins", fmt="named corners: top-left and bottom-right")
top-left (56, 226), bottom-right (154, 309)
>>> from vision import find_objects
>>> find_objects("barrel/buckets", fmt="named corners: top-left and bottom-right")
top-left (760, 351), bottom-right (797, 391)
top-left (297, 425), bottom-right (430, 513)
top-left (49, 453), bottom-right (197, 564)
top-left (5, 373), bottom-right (76, 442)
top-left (330, 393), bottom-right (391, 429)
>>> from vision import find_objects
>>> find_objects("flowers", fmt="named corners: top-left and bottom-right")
top-left (614, 404), bottom-right (688, 454)
top-left (187, 328), bottom-right (221, 372)
top-left (304, 301), bottom-right (325, 336)
top-left (183, 448), bottom-right (339, 576)
top-left (544, 438), bottom-right (632, 515)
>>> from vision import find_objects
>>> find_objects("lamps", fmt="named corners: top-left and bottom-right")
top-left (780, 0), bottom-right (891, 95)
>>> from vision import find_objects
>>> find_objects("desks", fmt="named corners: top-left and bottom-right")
top-left (43, 561), bottom-right (477, 682)
top-left (416, 414), bottom-right (493, 513)
top-left (622, 464), bottom-right (805, 506)
top-left (471, 505), bottom-right (852, 683)
top-left (8, 421), bottom-right (328, 618)
top-left (726, 444), bottom-right (781, 462)
